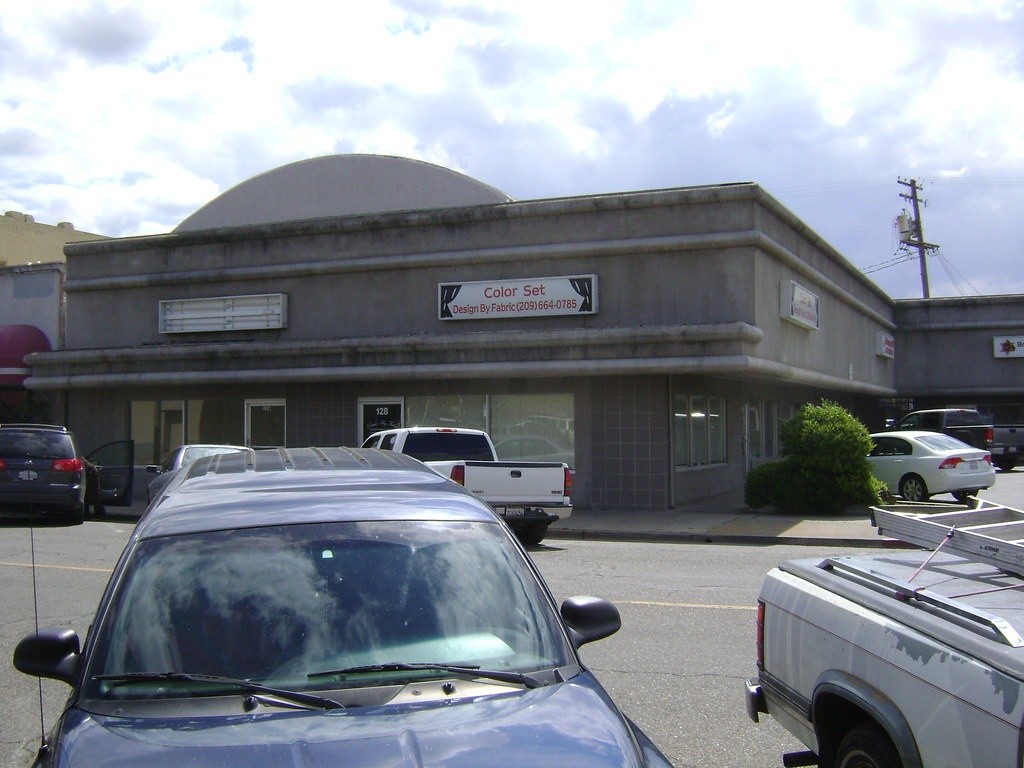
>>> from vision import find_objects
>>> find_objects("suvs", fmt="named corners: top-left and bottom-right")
top-left (0, 423), bottom-right (136, 526)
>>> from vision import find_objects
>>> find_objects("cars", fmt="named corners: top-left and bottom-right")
top-left (13, 444), bottom-right (689, 768)
top-left (867, 431), bottom-right (998, 501)
top-left (145, 444), bottom-right (255, 508)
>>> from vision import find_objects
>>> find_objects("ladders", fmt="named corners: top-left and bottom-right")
top-left (869, 495), bottom-right (1024, 576)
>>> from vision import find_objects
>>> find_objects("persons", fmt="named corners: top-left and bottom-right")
top-left (79, 456), bottom-right (106, 521)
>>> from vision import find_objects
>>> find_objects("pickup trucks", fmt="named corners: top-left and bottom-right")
top-left (360, 428), bottom-right (575, 544)
top-left (744, 527), bottom-right (1023, 768)
top-left (885, 409), bottom-right (1024, 472)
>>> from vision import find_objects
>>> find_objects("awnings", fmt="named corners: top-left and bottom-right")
top-left (0, 325), bottom-right (53, 385)
